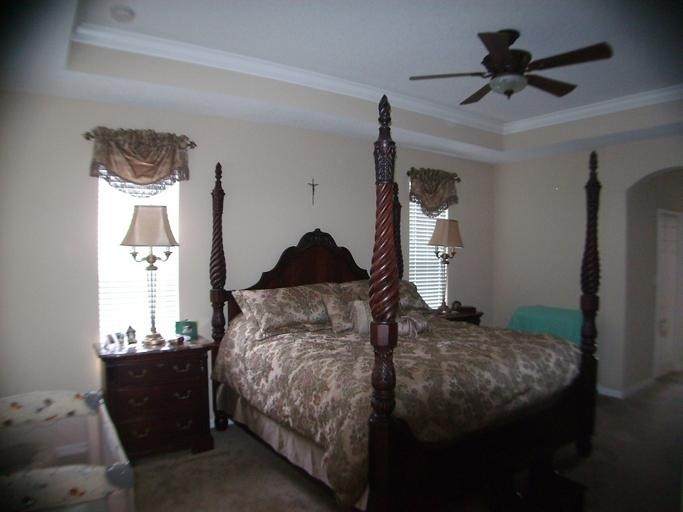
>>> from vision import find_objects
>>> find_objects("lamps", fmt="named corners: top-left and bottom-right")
top-left (491, 74), bottom-right (528, 100)
top-left (427, 218), bottom-right (464, 314)
top-left (120, 205), bottom-right (180, 346)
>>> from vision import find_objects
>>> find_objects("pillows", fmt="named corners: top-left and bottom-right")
top-left (231, 279), bottom-right (434, 343)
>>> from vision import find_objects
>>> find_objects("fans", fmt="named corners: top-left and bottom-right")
top-left (410, 29), bottom-right (613, 104)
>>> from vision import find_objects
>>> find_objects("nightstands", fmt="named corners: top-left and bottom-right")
top-left (92, 334), bottom-right (215, 466)
top-left (448, 312), bottom-right (484, 326)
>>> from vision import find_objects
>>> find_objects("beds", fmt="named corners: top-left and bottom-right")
top-left (209, 93), bottom-right (603, 511)
top-left (0, 390), bottom-right (135, 512)
